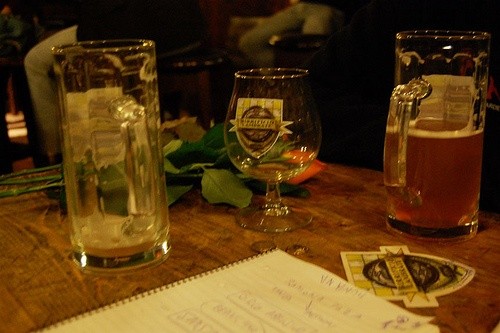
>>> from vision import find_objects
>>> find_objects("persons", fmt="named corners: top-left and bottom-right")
top-left (22, 0), bottom-right (202, 168)
top-left (237, 3), bottom-right (345, 84)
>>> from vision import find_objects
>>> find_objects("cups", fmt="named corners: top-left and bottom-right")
top-left (51, 37), bottom-right (174, 274)
top-left (384, 28), bottom-right (486, 246)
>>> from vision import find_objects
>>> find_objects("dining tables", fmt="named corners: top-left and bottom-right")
top-left (0, 164), bottom-right (500, 333)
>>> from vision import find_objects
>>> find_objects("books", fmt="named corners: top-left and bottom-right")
top-left (27, 245), bottom-right (441, 332)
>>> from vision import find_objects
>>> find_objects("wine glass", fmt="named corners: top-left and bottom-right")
top-left (223, 69), bottom-right (320, 235)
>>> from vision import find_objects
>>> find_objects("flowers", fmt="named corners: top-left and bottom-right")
top-left (0, 122), bottom-right (325, 210)
